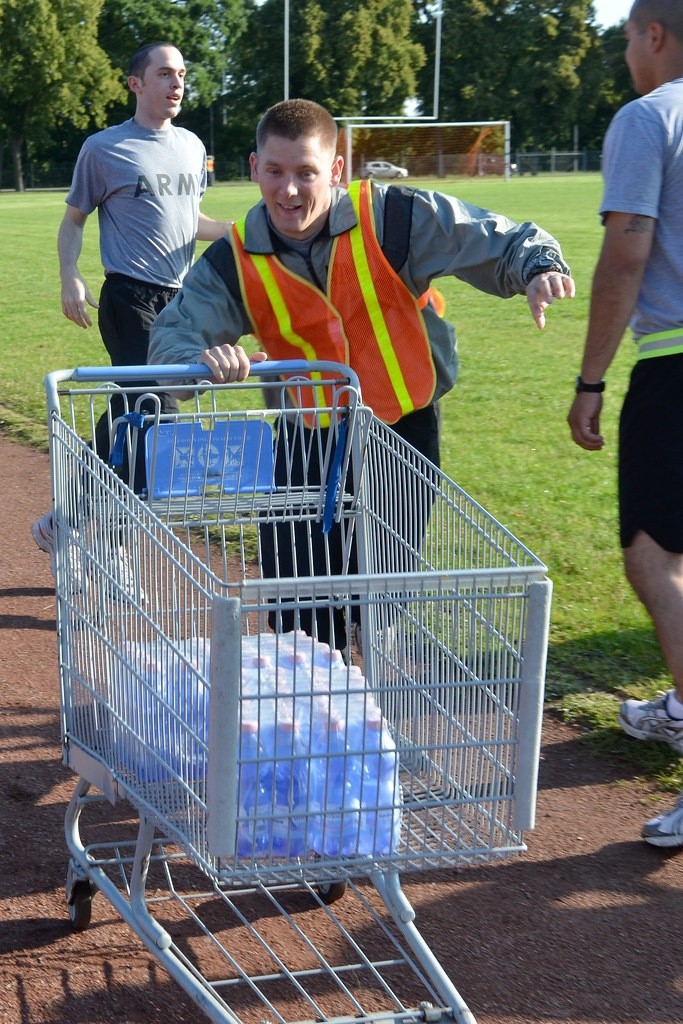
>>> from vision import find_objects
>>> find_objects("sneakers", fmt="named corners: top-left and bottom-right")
top-left (619, 691), bottom-right (683, 753)
top-left (87, 541), bottom-right (148, 607)
top-left (354, 622), bottom-right (394, 664)
top-left (31, 510), bottom-right (89, 593)
top-left (640, 793), bottom-right (683, 848)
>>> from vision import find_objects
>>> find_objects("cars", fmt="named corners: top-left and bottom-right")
top-left (452, 157), bottom-right (466, 175)
top-left (360, 159), bottom-right (408, 180)
top-left (466, 156), bottom-right (519, 176)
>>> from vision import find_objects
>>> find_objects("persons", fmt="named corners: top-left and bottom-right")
top-left (144, 98), bottom-right (575, 666)
top-left (565, 0), bottom-right (683, 848)
top-left (31, 41), bottom-right (236, 609)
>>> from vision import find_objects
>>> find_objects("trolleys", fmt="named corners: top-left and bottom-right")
top-left (42, 358), bottom-right (553, 1024)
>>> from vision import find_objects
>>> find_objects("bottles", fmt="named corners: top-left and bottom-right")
top-left (112, 630), bottom-right (401, 860)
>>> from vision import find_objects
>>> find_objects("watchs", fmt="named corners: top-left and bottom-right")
top-left (526, 259), bottom-right (563, 282)
top-left (575, 375), bottom-right (606, 394)
